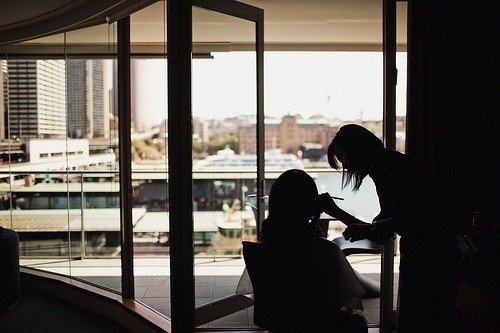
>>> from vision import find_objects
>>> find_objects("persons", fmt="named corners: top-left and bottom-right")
top-left (317, 125), bottom-right (450, 333)
top-left (257, 170), bottom-right (380, 333)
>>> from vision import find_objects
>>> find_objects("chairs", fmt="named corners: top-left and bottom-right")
top-left (331, 236), bottom-right (381, 298)
top-left (0, 226), bottom-right (21, 313)
top-left (242, 241), bottom-right (288, 333)
